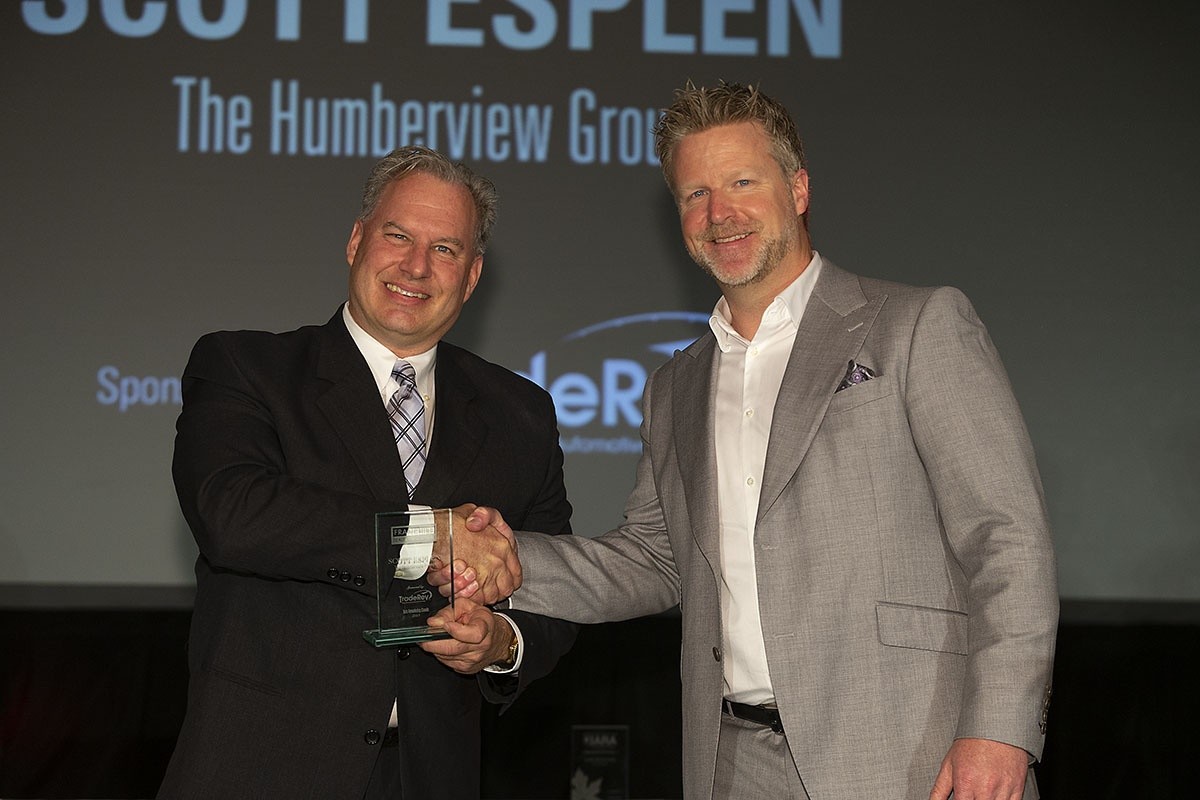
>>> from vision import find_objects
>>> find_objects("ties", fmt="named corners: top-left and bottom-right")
top-left (383, 361), bottom-right (427, 504)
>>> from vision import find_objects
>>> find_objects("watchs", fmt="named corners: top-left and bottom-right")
top-left (494, 613), bottom-right (519, 672)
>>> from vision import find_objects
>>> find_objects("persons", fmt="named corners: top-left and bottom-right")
top-left (432, 80), bottom-right (1061, 800)
top-left (155, 145), bottom-right (574, 800)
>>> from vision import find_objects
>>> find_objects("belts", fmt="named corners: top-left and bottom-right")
top-left (720, 697), bottom-right (785, 735)
